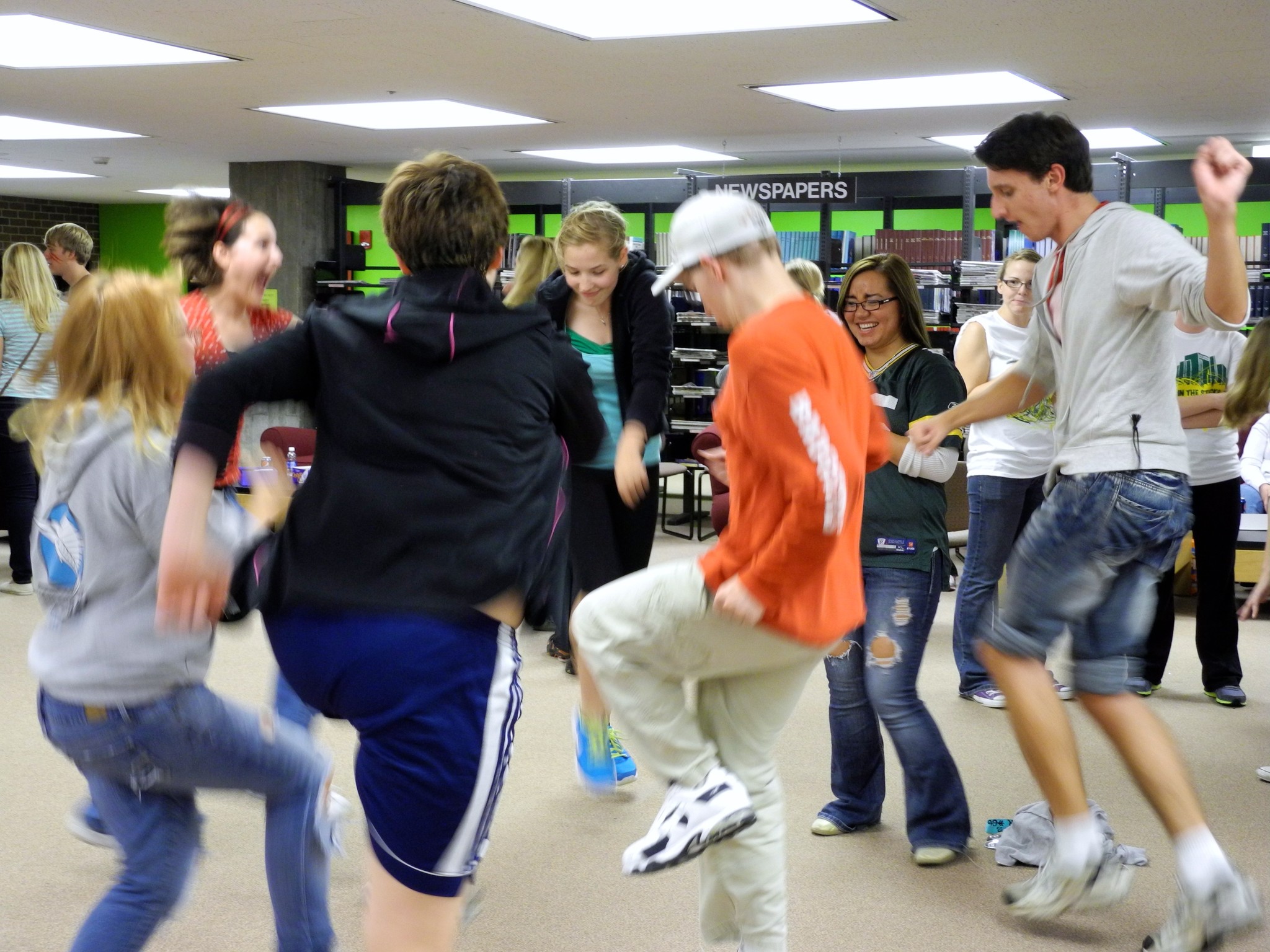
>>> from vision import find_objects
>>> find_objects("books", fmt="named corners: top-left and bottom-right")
top-left (626, 231), bottom-right (730, 434)
top-left (775, 229), bottom-right (1058, 355)
top-left (1183, 223), bottom-right (1270, 338)
top-left (499, 232), bottom-right (534, 288)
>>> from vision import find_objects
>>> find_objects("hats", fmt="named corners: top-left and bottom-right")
top-left (651, 189), bottom-right (776, 298)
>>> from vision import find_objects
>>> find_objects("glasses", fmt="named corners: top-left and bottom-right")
top-left (1001, 278), bottom-right (1032, 290)
top-left (842, 296), bottom-right (898, 312)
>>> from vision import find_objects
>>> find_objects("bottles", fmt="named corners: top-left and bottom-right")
top-left (287, 447), bottom-right (297, 477)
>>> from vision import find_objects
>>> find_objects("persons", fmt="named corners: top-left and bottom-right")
top-left (44, 223), bottom-right (94, 292)
top-left (31, 270), bottom-right (332, 951)
top-left (910, 109), bottom-right (1261, 952)
top-left (1224, 320), bottom-right (1270, 783)
top-left (785, 258), bottom-right (841, 325)
top-left (533, 203), bottom-right (674, 800)
top-left (954, 249), bottom-right (1072, 708)
top-left (73, 193), bottom-right (345, 843)
top-left (506, 234), bottom-right (575, 675)
top-left (1125, 308), bottom-right (1250, 706)
top-left (157, 150), bottom-right (606, 952)
top-left (570, 183), bottom-right (888, 952)
top-left (0, 242), bottom-right (71, 594)
top-left (809, 253), bottom-right (970, 863)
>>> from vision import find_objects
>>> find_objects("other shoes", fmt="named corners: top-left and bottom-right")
top-left (914, 846), bottom-right (955, 865)
top-left (0, 581), bottom-right (33, 595)
top-left (1142, 867), bottom-right (1257, 952)
top-left (67, 801), bottom-right (116, 849)
top-left (1256, 766), bottom-right (1270, 782)
top-left (999, 836), bottom-right (1130, 916)
top-left (811, 817), bottom-right (844, 836)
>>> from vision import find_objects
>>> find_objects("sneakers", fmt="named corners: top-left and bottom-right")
top-left (1204, 686), bottom-right (1246, 707)
top-left (967, 688), bottom-right (1007, 708)
top-left (573, 706), bottom-right (637, 786)
top-left (623, 767), bottom-right (758, 875)
top-left (1052, 679), bottom-right (1075, 700)
top-left (1128, 677), bottom-right (1162, 696)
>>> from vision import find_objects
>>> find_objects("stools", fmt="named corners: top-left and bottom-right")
top-left (658, 461), bottom-right (714, 541)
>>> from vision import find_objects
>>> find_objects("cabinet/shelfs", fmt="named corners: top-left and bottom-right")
top-left (320, 156), bottom-right (1270, 436)
